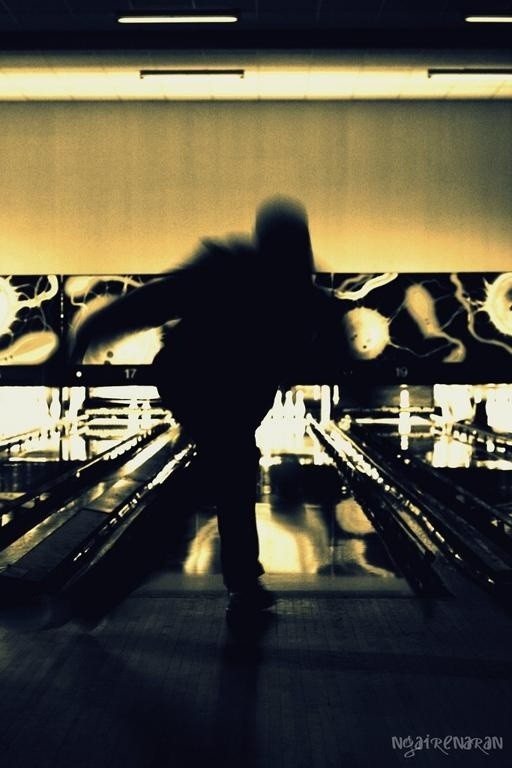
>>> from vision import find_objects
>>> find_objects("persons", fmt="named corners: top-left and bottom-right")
top-left (63, 194), bottom-right (355, 631)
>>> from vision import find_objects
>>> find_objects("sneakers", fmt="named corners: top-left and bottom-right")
top-left (224, 586), bottom-right (277, 614)
